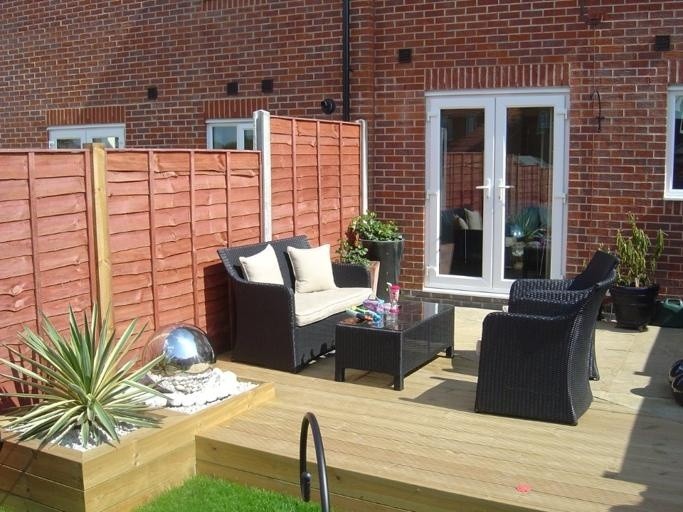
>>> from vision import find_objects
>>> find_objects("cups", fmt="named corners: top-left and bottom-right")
top-left (389, 285), bottom-right (400, 303)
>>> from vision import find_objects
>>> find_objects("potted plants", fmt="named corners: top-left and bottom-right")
top-left (347, 209), bottom-right (405, 301)
top-left (331, 239), bottom-right (380, 299)
top-left (597, 209), bottom-right (667, 333)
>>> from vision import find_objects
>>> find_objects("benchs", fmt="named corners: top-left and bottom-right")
top-left (217, 233), bottom-right (371, 373)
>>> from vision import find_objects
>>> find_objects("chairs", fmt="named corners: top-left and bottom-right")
top-left (509, 249), bottom-right (622, 380)
top-left (473, 267), bottom-right (619, 425)
